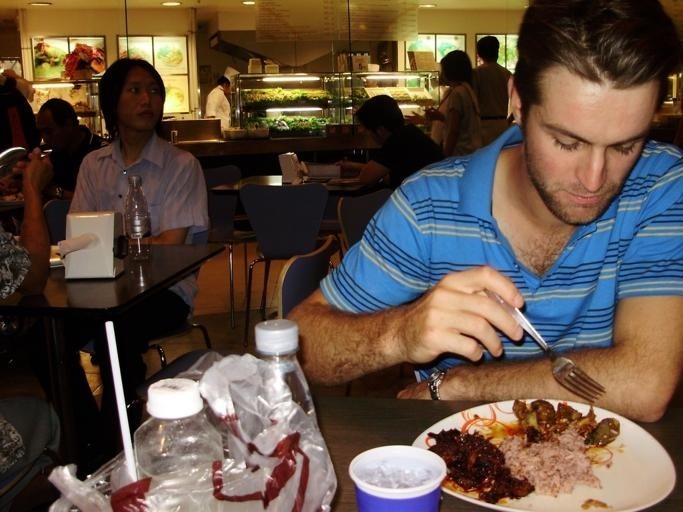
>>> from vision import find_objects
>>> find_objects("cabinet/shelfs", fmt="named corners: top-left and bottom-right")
top-left (231, 72), bottom-right (347, 136)
top-left (333, 70), bottom-right (443, 130)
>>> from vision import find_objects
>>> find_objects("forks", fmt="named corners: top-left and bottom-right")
top-left (483, 288), bottom-right (606, 403)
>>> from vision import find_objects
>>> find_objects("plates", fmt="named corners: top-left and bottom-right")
top-left (412, 399), bottom-right (677, 512)
top-left (50, 244), bottom-right (62, 266)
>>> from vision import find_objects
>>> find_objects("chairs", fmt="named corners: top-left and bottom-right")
top-left (206, 168), bottom-right (257, 329)
top-left (277, 243), bottom-right (327, 321)
top-left (0, 447), bottom-right (55, 512)
top-left (78, 322), bottom-right (212, 370)
top-left (241, 185), bottom-right (327, 339)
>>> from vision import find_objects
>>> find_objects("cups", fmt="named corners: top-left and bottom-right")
top-left (171, 131), bottom-right (177, 144)
top-left (349, 445), bottom-right (446, 512)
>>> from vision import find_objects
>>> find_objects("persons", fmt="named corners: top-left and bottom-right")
top-left (284, 0), bottom-right (683, 425)
top-left (401, 50), bottom-right (480, 158)
top-left (335, 95), bottom-right (447, 191)
top-left (203, 66), bottom-right (240, 138)
top-left (35, 98), bottom-right (110, 204)
top-left (34, 57), bottom-right (211, 451)
top-left (1, 148), bottom-right (60, 481)
top-left (473, 36), bottom-right (514, 148)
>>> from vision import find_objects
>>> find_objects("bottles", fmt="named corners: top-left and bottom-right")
top-left (133, 378), bottom-right (224, 492)
top-left (125, 176), bottom-right (152, 257)
top-left (238, 319), bottom-right (319, 440)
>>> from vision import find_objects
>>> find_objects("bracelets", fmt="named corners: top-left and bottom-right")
top-left (56, 186), bottom-right (63, 200)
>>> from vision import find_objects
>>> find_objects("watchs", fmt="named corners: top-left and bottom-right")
top-left (428, 367), bottom-right (449, 400)
